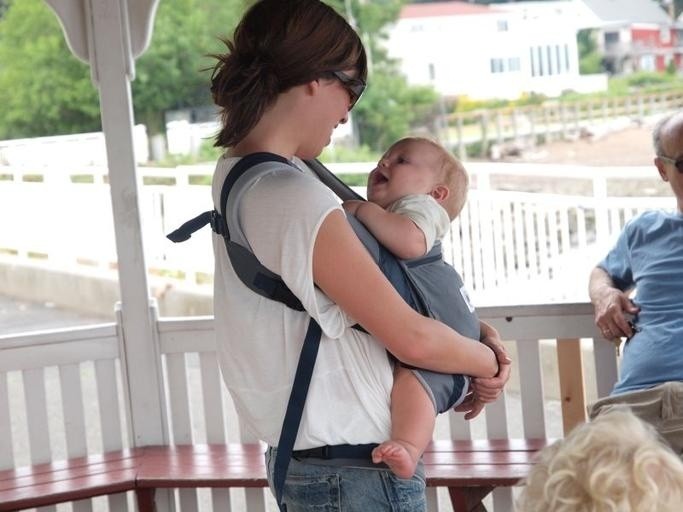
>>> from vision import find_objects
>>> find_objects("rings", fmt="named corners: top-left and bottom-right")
top-left (603, 329), bottom-right (609, 334)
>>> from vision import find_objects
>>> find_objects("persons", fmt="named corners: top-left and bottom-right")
top-left (340, 138), bottom-right (484, 479)
top-left (587, 109), bottom-right (683, 456)
top-left (515, 403), bottom-right (683, 511)
top-left (198, 0), bottom-right (513, 511)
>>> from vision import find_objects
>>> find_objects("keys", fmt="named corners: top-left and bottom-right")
top-left (614, 338), bottom-right (621, 355)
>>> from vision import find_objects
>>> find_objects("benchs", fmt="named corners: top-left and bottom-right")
top-left (0, 446), bottom-right (137, 512)
top-left (136, 442), bottom-right (565, 512)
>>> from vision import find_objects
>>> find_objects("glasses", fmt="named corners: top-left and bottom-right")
top-left (657, 150), bottom-right (683, 174)
top-left (333, 69), bottom-right (367, 111)
